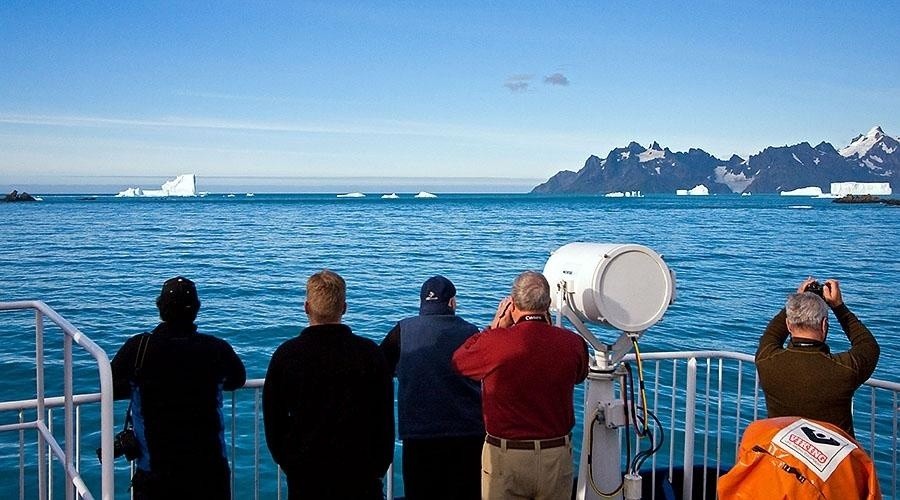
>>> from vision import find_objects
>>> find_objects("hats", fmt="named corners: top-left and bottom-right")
top-left (156, 276), bottom-right (198, 305)
top-left (420, 276), bottom-right (456, 314)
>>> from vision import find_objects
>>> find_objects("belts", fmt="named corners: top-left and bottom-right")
top-left (485, 432), bottom-right (572, 450)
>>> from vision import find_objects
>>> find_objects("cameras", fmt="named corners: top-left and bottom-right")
top-left (804, 281), bottom-right (827, 302)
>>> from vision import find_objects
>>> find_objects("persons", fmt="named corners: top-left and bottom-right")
top-left (755, 277), bottom-right (880, 441)
top-left (262, 272), bottom-right (396, 500)
top-left (92, 276), bottom-right (246, 500)
top-left (450, 271), bottom-right (590, 500)
top-left (376, 274), bottom-right (483, 500)
